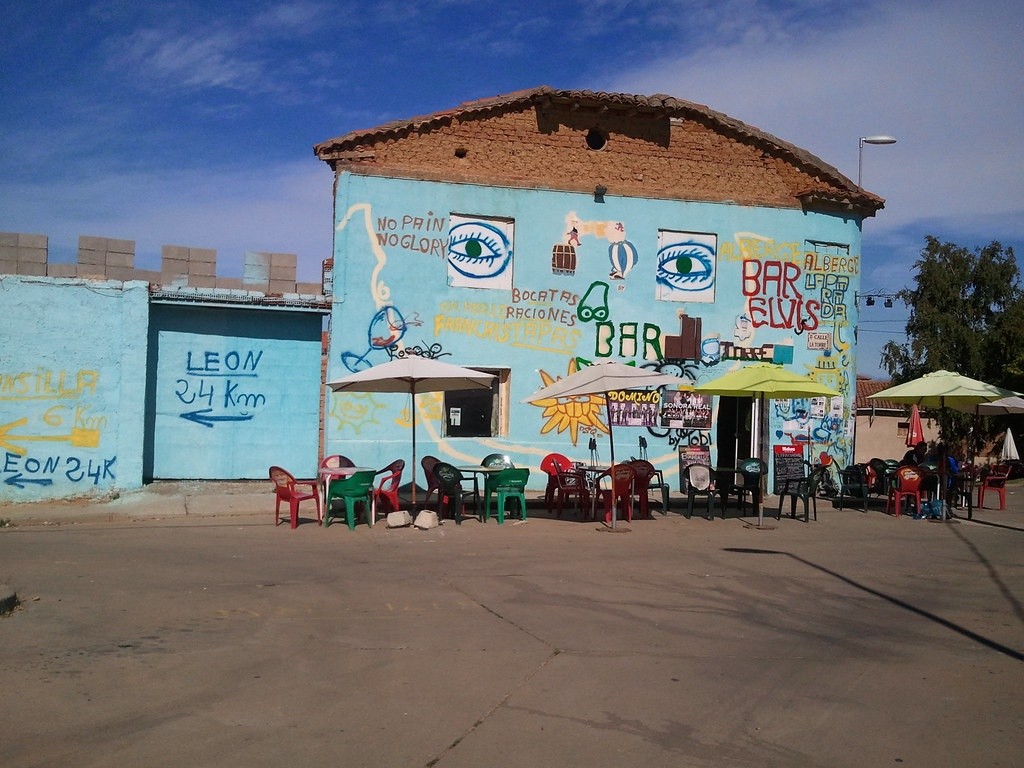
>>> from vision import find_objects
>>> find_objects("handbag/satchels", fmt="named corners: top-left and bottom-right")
top-left (926, 502), bottom-right (952, 520)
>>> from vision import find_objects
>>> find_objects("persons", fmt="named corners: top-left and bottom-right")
top-left (898, 442), bottom-right (929, 476)
top-left (931, 444), bottom-right (959, 507)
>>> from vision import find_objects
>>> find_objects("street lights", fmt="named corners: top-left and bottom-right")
top-left (858, 134), bottom-right (897, 188)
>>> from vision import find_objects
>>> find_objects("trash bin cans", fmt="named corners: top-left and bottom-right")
top-left (871, 457), bottom-right (899, 495)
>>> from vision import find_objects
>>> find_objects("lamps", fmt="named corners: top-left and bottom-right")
top-left (884, 298), bottom-right (892, 308)
top-left (866, 297), bottom-right (875, 306)
top-left (858, 135), bottom-right (896, 187)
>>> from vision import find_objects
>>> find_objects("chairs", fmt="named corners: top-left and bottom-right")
top-left (839, 458), bottom-right (1012, 518)
top-left (476, 455), bottom-right (516, 507)
top-left (551, 457), bottom-right (589, 518)
top-left (593, 465), bottom-right (637, 523)
top-left (432, 462), bottom-right (482, 525)
top-left (269, 466), bottom-right (322, 529)
top-left (369, 459), bottom-right (405, 519)
top-left (777, 466), bottom-right (826, 523)
top-left (647, 469), bottom-right (669, 512)
top-left (320, 455), bottom-right (357, 518)
top-left (324, 470), bottom-right (376, 530)
top-left (728, 458), bottom-right (768, 515)
top-left (683, 463), bottom-right (716, 520)
top-left (626, 459), bottom-right (655, 519)
top-left (540, 453), bottom-right (578, 513)
top-left (421, 455), bottom-right (464, 516)
top-left (485, 468), bottom-right (530, 524)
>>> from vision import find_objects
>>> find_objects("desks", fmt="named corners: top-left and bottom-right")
top-left (318, 465), bottom-right (375, 525)
top-left (577, 466), bottom-right (635, 519)
top-left (456, 465), bottom-right (528, 515)
top-left (682, 466), bottom-right (742, 519)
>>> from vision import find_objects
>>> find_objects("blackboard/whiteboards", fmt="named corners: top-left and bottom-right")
top-left (676, 444), bottom-right (711, 494)
top-left (774, 444), bottom-right (807, 495)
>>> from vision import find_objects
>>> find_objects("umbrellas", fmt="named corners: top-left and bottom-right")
top-left (696, 365), bottom-right (843, 459)
top-left (866, 370), bottom-right (1024, 519)
top-left (520, 362), bottom-right (695, 528)
top-left (325, 355), bottom-right (497, 503)
top-left (961, 397), bottom-right (1024, 416)
top-left (1002, 426), bottom-right (1019, 460)
top-left (905, 405), bottom-right (924, 447)
